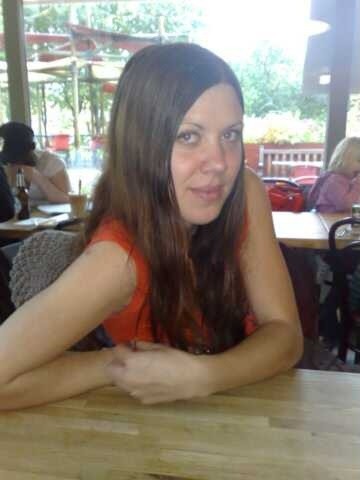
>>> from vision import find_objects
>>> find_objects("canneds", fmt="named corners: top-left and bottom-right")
top-left (351, 202), bottom-right (360, 228)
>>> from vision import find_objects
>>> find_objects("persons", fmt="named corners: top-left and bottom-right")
top-left (0, 115), bottom-right (73, 204)
top-left (0, 166), bottom-right (22, 262)
top-left (308, 138), bottom-right (360, 212)
top-left (0, 43), bottom-right (304, 412)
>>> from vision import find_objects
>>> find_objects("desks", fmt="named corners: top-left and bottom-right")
top-left (0, 353), bottom-right (360, 480)
top-left (91, 136), bottom-right (110, 168)
top-left (258, 161), bottom-right (323, 177)
top-left (1, 191), bottom-right (360, 250)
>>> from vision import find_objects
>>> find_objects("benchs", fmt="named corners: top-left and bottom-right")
top-left (257, 144), bottom-right (324, 178)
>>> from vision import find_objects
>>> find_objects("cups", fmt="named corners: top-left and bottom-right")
top-left (67, 192), bottom-right (87, 218)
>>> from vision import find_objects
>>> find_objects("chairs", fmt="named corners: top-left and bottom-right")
top-left (45, 134), bottom-right (71, 169)
top-left (318, 219), bottom-right (360, 367)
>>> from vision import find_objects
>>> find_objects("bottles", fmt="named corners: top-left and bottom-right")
top-left (13, 168), bottom-right (30, 220)
